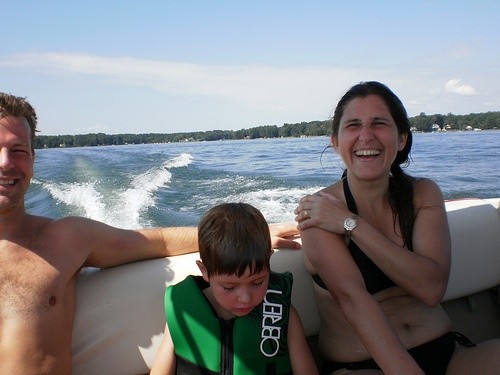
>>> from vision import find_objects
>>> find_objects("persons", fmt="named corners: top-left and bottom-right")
top-left (293, 82), bottom-right (500, 375)
top-left (148, 203), bottom-right (320, 375)
top-left (1, 93), bottom-right (301, 375)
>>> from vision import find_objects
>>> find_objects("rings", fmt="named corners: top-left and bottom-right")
top-left (304, 210), bottom-right (308, 218)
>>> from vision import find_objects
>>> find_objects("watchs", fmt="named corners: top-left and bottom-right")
top-left (343, 214), bottom-right (365, 239)
